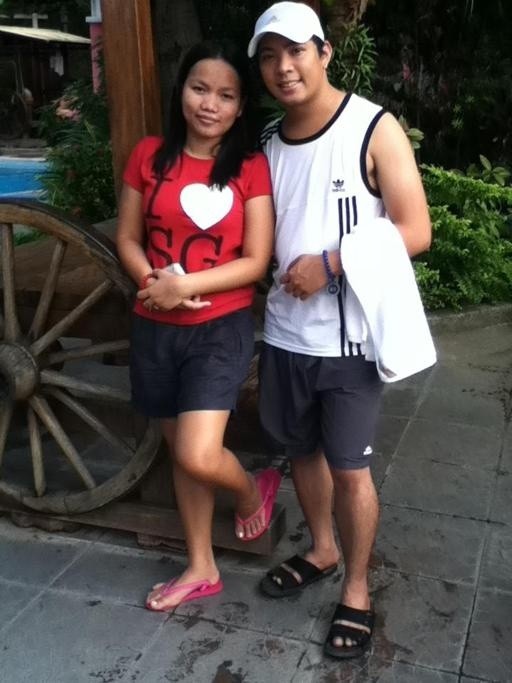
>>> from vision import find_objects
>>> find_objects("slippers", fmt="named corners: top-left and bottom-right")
top-left (144, 568), bottom-right (223, 614)
top-left (324, 598), bottom-right (373, 660)
top-left (257, 555), bottom-right (340, 600)
top-left (235, 466), bottom-right (281, 542)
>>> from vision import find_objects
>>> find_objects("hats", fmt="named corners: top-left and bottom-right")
top-left (246, 2), bottom-right (326, 59)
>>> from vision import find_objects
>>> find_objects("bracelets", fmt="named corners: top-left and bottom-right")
top-left (136, 271), bottom-right (156, 288)
top-left (319, 247), bottom-right (337, 281)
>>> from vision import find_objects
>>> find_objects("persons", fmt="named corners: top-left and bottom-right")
top-left (107, 36), bottom-right (283, 615)
top-left (242, 1), bottom-right (434, 664)
